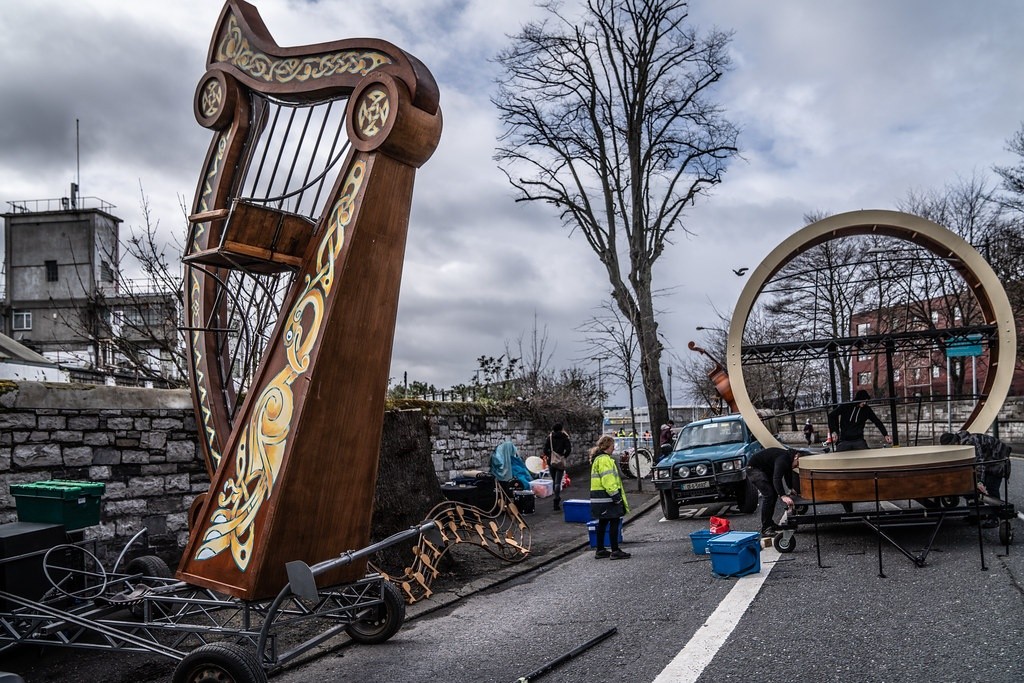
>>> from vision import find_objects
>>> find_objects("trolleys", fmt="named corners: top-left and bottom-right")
top-left (0, 518), bottom-right (438, 683)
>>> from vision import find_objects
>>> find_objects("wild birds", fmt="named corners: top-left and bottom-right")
top-left (734, 268), bottom-right (748, 277)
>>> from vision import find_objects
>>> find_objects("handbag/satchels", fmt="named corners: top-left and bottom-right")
top-left (550, 451), bottom-right (566, 469)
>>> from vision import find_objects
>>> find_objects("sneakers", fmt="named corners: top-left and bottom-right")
top-left (595, 548), bottom-right (611, 559)
top-left (610, 548), bottom-right (631, 560)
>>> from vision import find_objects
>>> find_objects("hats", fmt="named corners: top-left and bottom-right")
top-left (661, 424), bottom-right (670, 430)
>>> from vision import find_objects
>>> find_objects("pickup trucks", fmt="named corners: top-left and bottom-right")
top-left (650, 408), bottom-right (781, 520)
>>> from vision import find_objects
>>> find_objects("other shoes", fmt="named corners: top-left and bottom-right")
top-left (762, 527), bottom-right (777, 537)
top-left (773, 523), bottom-right (784, 531)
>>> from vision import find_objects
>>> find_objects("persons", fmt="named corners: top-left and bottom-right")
top-left (796, 401), bottom-right (812, 409)
top-left (803, 418), bottom-right (815, 445)
top-left (828, 390), bottom-right (893, 453)
top-left (643, 430), bottom-right (651, 448)
top-left (940, 430), bottom-right (1007, 529)
top-left (587, 436), bottom-right (631, 560)
top-left (629, 429), bottom-right (638, 448)
top-left (543, 422), bottom-right (572, 511)
top-left (615, 428), bottom-right (627, 449)
top-left (656, 420), bottom-right (675, 462)
top-left (746, 447), bottom-right (809, 537)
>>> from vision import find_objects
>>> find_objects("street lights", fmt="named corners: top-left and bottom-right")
top-left (668, 367), bottom-right (672, 406)
top-left (866, 232), bottom-right (999, 439)
top-left (591, 356), bottom-right (608, 408)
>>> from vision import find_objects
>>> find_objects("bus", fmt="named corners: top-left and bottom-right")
top-left (604, 417), bottom-right (632, 424)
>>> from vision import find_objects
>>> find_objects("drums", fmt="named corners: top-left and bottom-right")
top-left (620, 445), bottom-right (653, 479)
top-left (799, 445), bottom-right (976, 502)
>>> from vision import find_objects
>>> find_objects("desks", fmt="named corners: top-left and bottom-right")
top-left (442, 481), bottom-right (513, 517)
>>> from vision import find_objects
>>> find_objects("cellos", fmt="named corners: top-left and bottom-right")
top-left (688, 341), bottom-right (762, 436)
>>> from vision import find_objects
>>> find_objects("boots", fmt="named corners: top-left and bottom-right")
top-left (554, 499), bottom-right (561, 511)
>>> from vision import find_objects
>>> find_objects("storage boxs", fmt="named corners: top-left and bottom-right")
top-left (8, 480), bottom-right (109, 530)
top-left (708, 531), bottom-right (763, 577)
top-left (563, 500), bottom-right (594, 522)
top-left (690, 529), bottom-right (724, 554)
top-left (588, 518), bottom-right (624, 548)
top-left (531, 479), bottom-right (554, 499)
